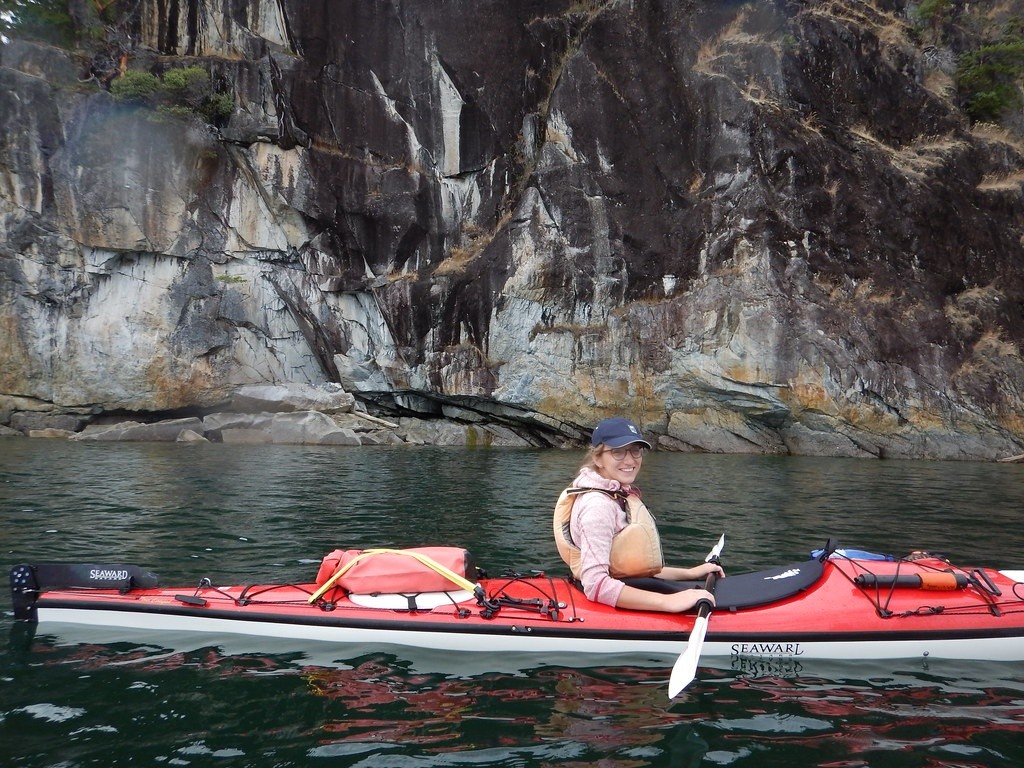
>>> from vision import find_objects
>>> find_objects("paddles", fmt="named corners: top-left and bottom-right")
top-left (667, 532), bottom-right (725, 700)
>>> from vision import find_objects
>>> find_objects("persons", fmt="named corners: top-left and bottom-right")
top-left (569, 417), bottom-right (726, 613)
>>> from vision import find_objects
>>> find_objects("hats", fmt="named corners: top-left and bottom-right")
top-left (592, 418), bottom-right (652, 450)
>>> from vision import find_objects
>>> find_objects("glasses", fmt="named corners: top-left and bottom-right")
top-left (598, 445), bottom-right (642, 461)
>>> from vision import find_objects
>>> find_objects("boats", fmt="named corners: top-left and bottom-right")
top-left (6, 544), bottom-right (1024, 684)
